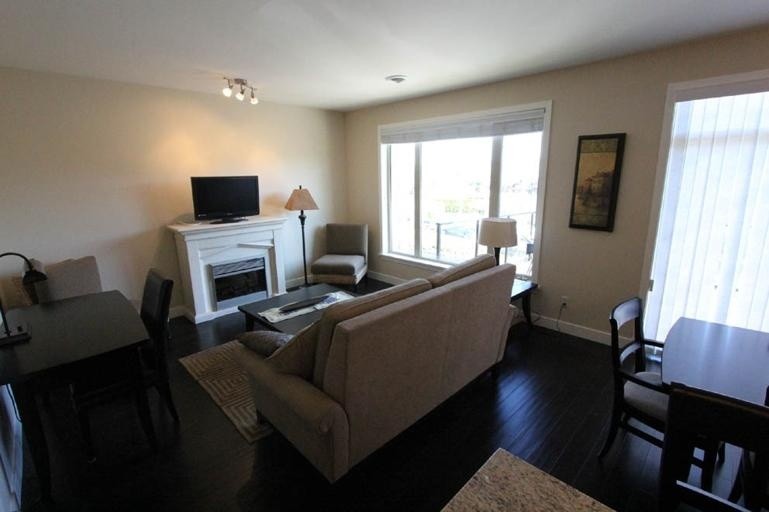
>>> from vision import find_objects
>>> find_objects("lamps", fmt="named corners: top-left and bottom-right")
top-left (285, 186), bottom-right (319, 289)
top-left (223, 78), bottom-right (258, 105)
top-left (0, 252), bottom-right (48, 347)
top-left (480, 219), bottom-right (518, 265)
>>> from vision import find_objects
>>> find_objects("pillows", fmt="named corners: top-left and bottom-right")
top-left (236, 330), bottom-right (294, 356)
top-left (267, 317), bottom-right (319, 373)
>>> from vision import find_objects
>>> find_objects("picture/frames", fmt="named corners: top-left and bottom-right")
top-left (569, 132), bottom-right (626, 232)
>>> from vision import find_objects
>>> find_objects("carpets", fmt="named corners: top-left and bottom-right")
top-left (180, 341), bottom-right (273, 443)
top-left (441, 448), bottom-right (616, 512)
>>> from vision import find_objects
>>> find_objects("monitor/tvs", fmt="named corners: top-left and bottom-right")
top-left (191, 176), bottom-right (259, 224)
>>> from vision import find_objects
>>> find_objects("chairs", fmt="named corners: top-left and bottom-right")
top-left (729, 448), bottom-right (769, 512)
top-left (312, 222), bottom-right (368, 284)
top-left (599, 298), bottom-right (723, 492)
top-left (70, 268), bottom-right (180, 446)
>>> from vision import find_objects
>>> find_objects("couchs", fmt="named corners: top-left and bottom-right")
top-left (0, 256), bottom-right (102, 312)
top-left (238, 254), bottom-right (515, 484)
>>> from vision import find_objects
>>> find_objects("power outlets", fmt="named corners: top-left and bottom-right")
top-left (560, 296), bottom-right (568, 310)
